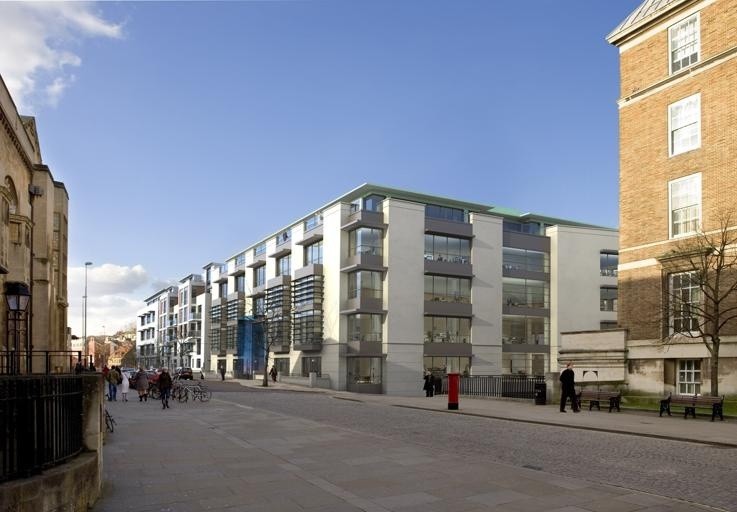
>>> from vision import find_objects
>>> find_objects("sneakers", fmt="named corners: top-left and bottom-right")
top-left (560, 408), bottom-right (579, 413)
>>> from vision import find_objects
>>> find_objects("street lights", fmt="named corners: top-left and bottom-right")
top-left (84, 261), bottom-right (92, 369)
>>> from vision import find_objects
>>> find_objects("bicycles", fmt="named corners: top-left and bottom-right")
top-left (148, 381), bottom-right (211, 403)
top-left (105, 410), bottom-right (117, 432)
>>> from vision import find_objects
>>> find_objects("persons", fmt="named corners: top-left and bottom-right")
top-left (434, 374), bottom-right (442, 395)
top-left (220, 365), bottom-right (226, 380)
top-left (424, 371), bottom-right (434, 397)
top-left (50, 360), bottom-right (172, 408)
top-left (270, 365), bottom-right (277, 381)
top-left (559, 363), bottom-right (581, 412)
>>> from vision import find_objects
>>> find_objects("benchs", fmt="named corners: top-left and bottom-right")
top-left (570, 391), bottom-right (621, 412)
top-left (659, 392), bottom-right (725, 422)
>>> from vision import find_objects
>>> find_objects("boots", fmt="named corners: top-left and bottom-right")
top-left (139, 394), bottom-right (147, 401)
top-left (163, 401), bottom-right (170, 409)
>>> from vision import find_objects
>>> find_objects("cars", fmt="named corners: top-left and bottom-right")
top-left (175, 367), bottom-right (193, 380)
top-left (120, 367), bottom-right (162, 389)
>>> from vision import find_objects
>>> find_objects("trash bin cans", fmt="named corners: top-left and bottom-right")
top-left (534, 383), bottom-right (546, 405)
